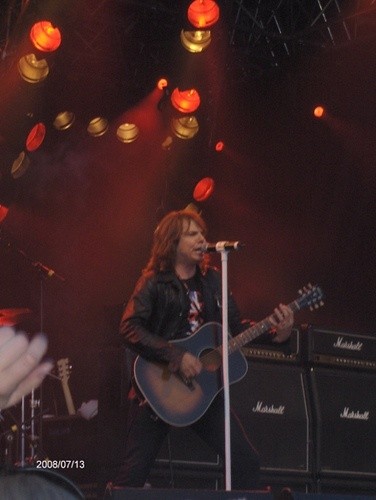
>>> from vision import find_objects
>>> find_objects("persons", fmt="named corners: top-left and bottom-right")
top-left (0, 327), bottom-right (53, 410)
top-left (117, 212), bottom-right (295, 489)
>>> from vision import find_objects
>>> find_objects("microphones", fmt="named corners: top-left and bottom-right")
top-left (201, 240), bottom-right (241, 254)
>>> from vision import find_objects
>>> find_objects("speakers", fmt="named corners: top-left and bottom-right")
top-left (300, 330), bottom-right (376, 478)
top-left (112, 321), bottom-right (312, 476)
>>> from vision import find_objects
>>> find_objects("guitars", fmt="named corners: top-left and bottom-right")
top-left (133, 283), bottom-right (324, 427)
top-left (57, 358), bottom-right (76, 415)
